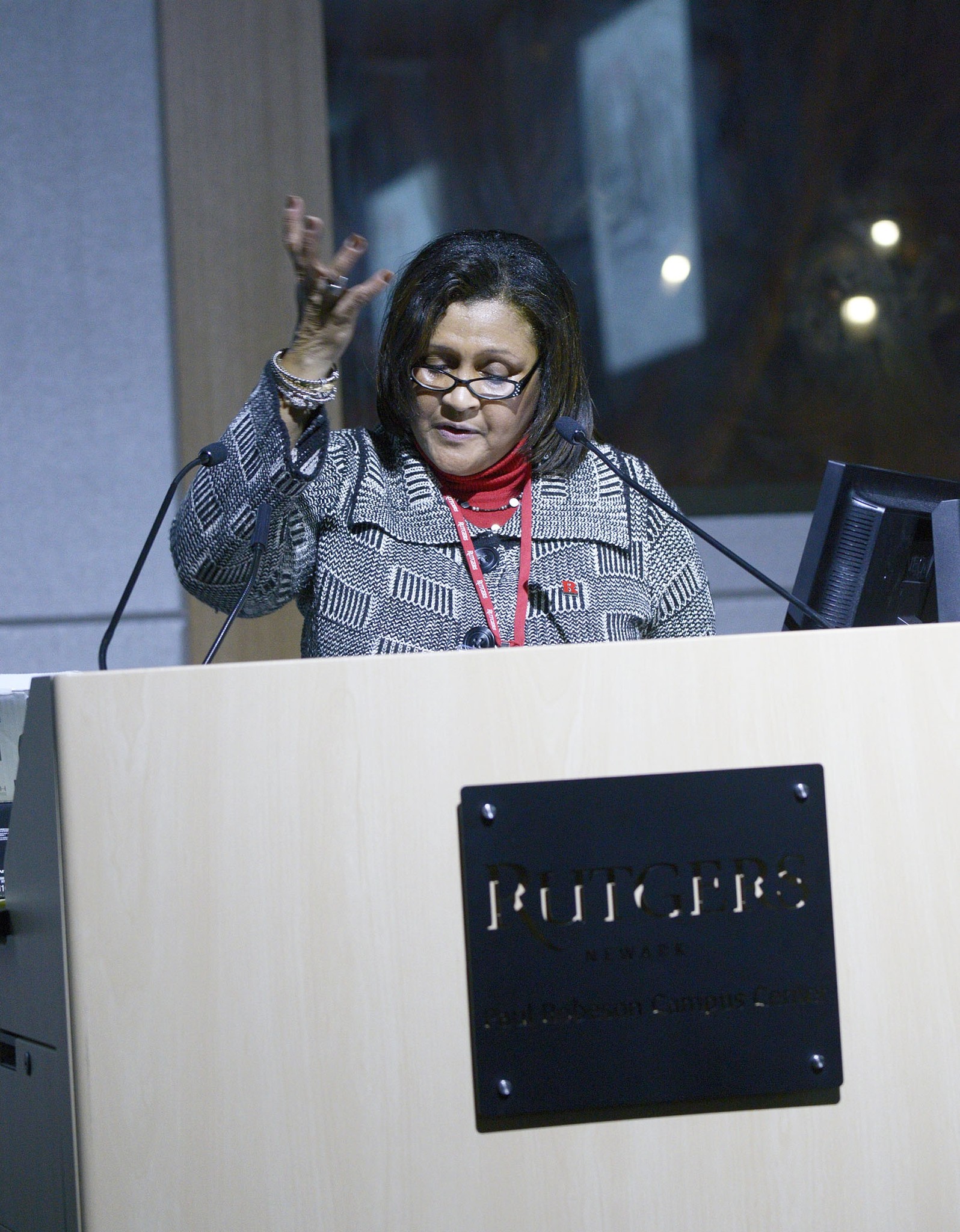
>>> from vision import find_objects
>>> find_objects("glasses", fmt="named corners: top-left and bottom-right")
top-left (409, 355), bottom-right (544, 403)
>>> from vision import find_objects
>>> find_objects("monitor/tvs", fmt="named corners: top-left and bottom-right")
top-left (781, 459), bottom-right (960, 632)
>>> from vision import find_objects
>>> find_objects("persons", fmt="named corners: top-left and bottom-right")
top-left (166, 193), bottom-right (715, 660)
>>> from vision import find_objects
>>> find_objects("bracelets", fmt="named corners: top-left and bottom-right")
top-left (271, 348), bottom-right (340, 415)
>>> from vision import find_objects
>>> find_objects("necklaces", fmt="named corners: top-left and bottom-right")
top-left (447, 489), bottom-right (524, 512)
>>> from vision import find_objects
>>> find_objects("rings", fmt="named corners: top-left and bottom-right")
top-left (326, 273), bottom-right (347, 298)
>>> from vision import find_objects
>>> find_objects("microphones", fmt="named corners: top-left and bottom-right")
top-left (201, 501), bottom-right (270, 666)
top-left (555, 415), bottom-right (837, 630)
top-left (96, 439), bottom-right (229, 672)
